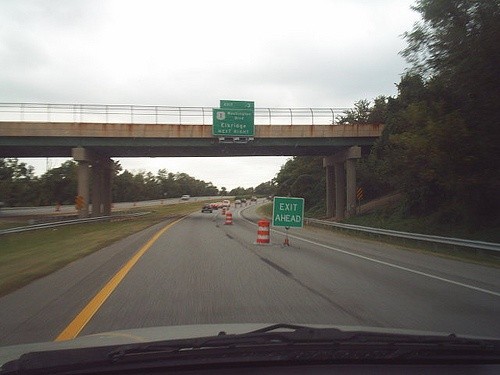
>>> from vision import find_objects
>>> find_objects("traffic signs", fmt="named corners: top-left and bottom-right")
top-left (272, 196), bottom-right (304, 228)
top-left (213, 101), bottom-right (254, 137)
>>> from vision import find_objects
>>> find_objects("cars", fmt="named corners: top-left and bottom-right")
top-left (201, 195), bottom-right (257, 213)
top-left (180, 194), bottom-right (190, 200)
